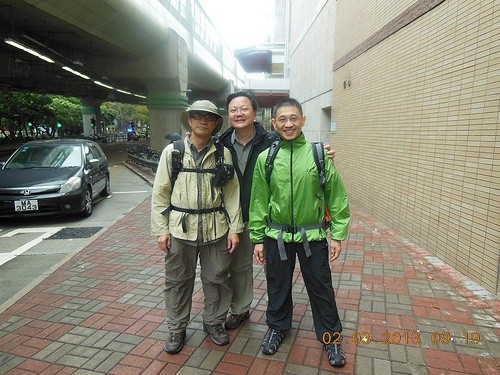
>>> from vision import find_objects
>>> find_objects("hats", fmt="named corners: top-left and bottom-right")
top-left (181, 100), bottom-right (223, 136)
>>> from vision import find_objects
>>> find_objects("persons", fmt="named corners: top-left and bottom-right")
top-left (151, 100), bottom-right (246, 352)
top-left (249, 97), bottom-right (351, 366)
top-left (219, 93), bottom-right (336, 330)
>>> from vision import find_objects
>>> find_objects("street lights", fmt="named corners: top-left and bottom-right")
top-left (131, 121), bottom-right (135, 146)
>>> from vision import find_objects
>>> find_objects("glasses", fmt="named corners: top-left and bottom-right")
top-left (190, 113), bottom-right (217, 121)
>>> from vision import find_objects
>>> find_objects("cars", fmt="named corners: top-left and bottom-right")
top-left (0, 137), bottom-right (112, 220)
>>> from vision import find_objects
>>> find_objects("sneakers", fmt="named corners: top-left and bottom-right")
top-left (261, 327), bottom-right (288, 354)
top-left (202, 322), bottom-right (230, 345)
top-left (225, 310), bottom-right (250, 329)
top-left (325, 342), bottom-right (346, 366)
top-left (165, 327), bottom-right (186, 353)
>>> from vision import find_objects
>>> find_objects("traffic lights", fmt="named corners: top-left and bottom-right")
top-left (57, 122), bottom-right (62, 128)
top-left (27, 122), bottom-right (34, 129)
top-left (127, 128), bottom-right (132, 132)
top-left (91, 119), bottom-right (95, 129)
